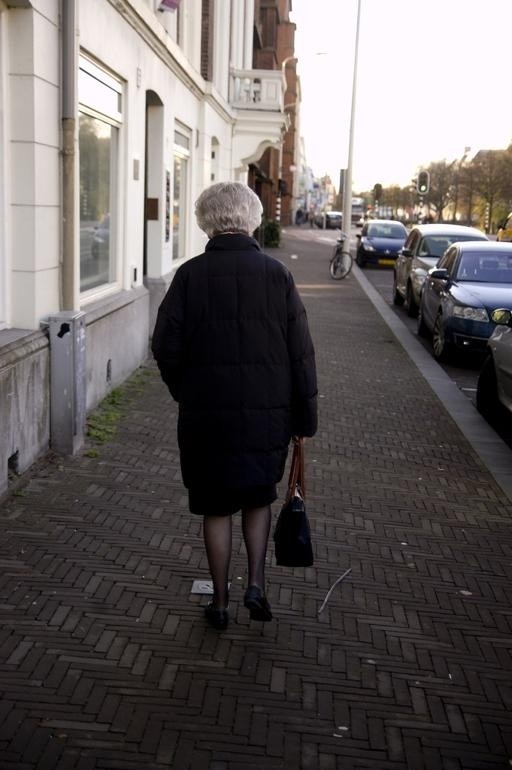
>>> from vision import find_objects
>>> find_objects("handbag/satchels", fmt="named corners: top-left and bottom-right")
top-left (270, 489), bottom-right (318, 571)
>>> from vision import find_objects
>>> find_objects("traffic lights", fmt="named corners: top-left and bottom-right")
top-left (418, 170), bottom-right (430, 195)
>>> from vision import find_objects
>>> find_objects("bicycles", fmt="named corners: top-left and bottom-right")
top-left (328, 227), bottom-right (354, 283)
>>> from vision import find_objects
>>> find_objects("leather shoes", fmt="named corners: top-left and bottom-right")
top-left (203, 600), bottom-right (231, 631)
top-left (242, 583), bottom-right (274, 623)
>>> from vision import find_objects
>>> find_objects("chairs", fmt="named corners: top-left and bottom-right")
top-left (460, 255), bottom-right (512, 282)
top-left (370, 227), bottom-right (402, 237)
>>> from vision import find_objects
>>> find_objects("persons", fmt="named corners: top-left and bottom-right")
top-left (151, 181), bottom-right (320, 629)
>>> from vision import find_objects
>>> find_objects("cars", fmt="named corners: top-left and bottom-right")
top-left (355, 212), bottom-right (512, 438)
top-left (314, 211), bottom-right (344, 228)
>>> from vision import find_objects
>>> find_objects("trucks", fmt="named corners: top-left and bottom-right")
top-left (350, 198), bottom-right (364, 224)
